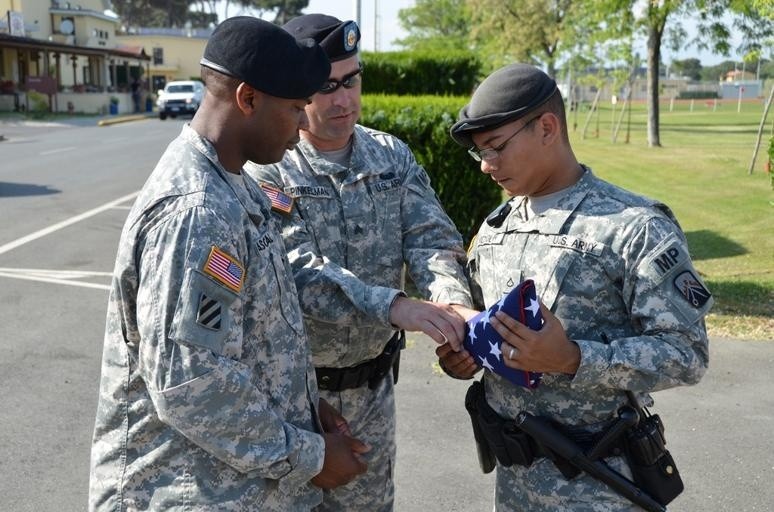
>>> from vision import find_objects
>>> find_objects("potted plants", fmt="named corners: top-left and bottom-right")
top-left (146, 95), bottom-right (152, 112)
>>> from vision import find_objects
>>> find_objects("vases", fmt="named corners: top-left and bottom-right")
top-left (110, 97), bottom-right (118, 114)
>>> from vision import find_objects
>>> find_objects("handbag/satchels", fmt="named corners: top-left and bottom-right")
top-left (622, 421), bottom-right (684, 506)
top-left (465, 380), bottom-right (534, 474)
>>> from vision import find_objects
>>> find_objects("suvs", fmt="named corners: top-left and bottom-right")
top-left (154, 79), bottom-right (206, 119)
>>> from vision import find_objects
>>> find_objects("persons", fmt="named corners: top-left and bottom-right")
top-left (87, 16), bottom-right (372, 511)
top-left (242, 15), bottom-right (480, 511)
top-left (435, 64), bottom-right (709, 511)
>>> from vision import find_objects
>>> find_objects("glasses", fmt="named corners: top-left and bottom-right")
top-left (468, 115), bottom-right (541, 162)
top-left (317, 66), bottom-right (364, 95)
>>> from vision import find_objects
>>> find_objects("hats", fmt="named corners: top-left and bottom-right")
top-left (200, 13), bottom-right (361, 99)
top-left (450, 64), bottom-right (557, 147)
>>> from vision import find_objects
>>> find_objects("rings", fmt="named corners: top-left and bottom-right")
top-left (508, 348), bottom-right (514, 360)
